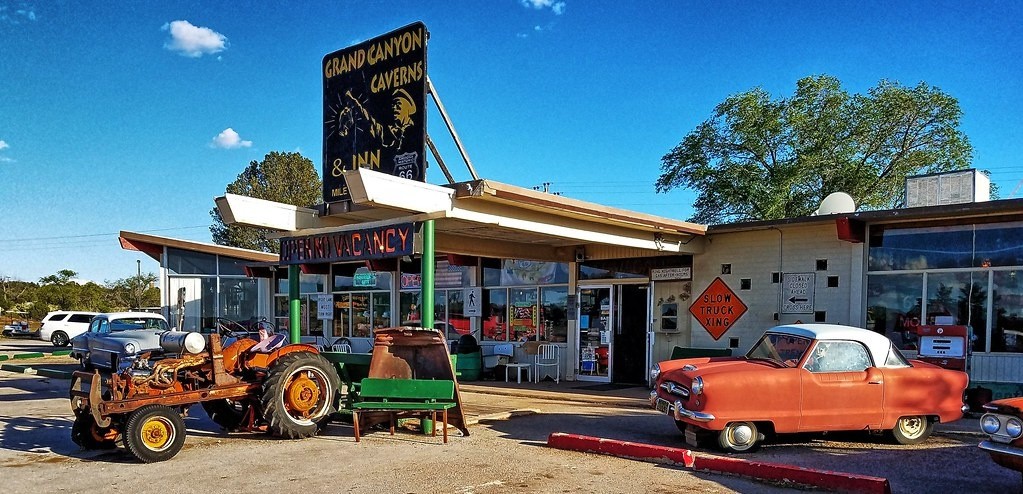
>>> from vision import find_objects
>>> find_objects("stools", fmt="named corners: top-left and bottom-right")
top-left (505, 363), bottom-right (532, 384)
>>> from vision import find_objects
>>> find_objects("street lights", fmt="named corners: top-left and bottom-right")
top-left (137, 260), bottom-right (141, 312)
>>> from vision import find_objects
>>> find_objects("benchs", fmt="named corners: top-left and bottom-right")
top-left (350, 377), bottom-right (456, 443)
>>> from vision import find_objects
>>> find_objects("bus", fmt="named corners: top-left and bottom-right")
top-left (410, 288), bottom-right (544, 341)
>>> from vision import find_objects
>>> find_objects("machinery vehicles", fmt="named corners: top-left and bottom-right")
top-left (67, 317), bottom-right (343, 464)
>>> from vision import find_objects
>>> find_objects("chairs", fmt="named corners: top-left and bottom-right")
top-left (534, 344), bottom-right (560, 384)
top-left (331, 338), bottom-right (351, 347)
top-left (331, 344), bottom-right (352, 353)
top-left (250, 334), bottom-right (286, 353)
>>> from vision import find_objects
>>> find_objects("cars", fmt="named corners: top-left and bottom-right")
top-left (39, 310), bottom-right (142, 347)
top-left (648, 323), bottom-right (971, 453)
top-left (403, 320), bottom-right (460, 340)
top-left (70, 312), bottom-right (177, 373)
top-left (977, 396), bottom-right (1023, 472)
